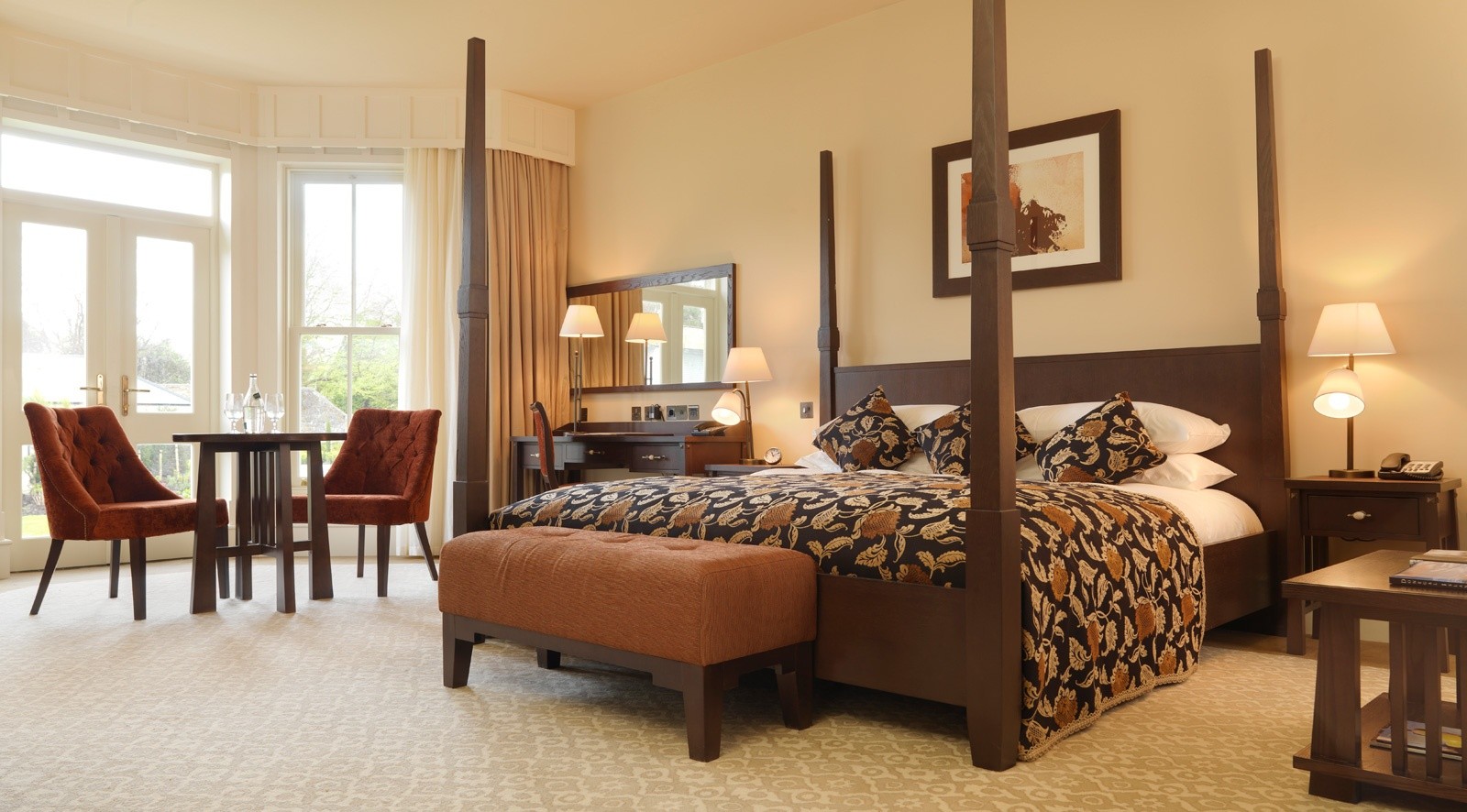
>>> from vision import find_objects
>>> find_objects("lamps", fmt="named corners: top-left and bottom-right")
top-left (1304, 300), bottom-right (1396, 480)
top-left (557, 302), bottom-right (605, 435)
top-left (709, 344), bottom-right (775, 466)
top-left (624, 312), bottom-right (669, 385)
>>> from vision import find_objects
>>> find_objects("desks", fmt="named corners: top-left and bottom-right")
top-left (166, 428), bottom-right (351, 616)
top-left (505, 420), bottom-right (750, 504)
top-left (1278, 547), bottom-right (1467, 812)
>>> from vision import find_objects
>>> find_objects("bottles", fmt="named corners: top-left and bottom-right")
top-left (242, 373), bottom-right (265, 435)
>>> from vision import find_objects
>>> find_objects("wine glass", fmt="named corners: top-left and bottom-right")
top-left (224, 393), bottom-right (244, 434)
top-left (264, 391), bottom-right (286, 434)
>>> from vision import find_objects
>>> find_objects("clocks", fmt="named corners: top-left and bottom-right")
top-left (763, 447), bottom-right (783, 465)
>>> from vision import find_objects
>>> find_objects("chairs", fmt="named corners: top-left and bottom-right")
top-left (265, 404), bottom-right (440, 601)
top-left (526, 400), bottom-right (586, 490)
top-left (18, 393), bottom-right (234, 625)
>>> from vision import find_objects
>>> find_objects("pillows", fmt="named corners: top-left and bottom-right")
top-left (795, 448), bottom-right (932, 474)
top-left (911, 400), bottom-right (1039, 475)
top-left (1018, 452), bottom-right (1238, 494)
top-left (812, 382), bottom-right (923, 472)
top-left (812, 401), bottom-right (962, 441)
top-left (1033, 389), bottom-right (1167, 490)
top-left (1016, 399), bottom-right (1234, 454)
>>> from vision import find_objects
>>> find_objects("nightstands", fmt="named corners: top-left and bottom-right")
top-left (701, 462), bottom-right (808, 476)
top-left (1284, 470), bottom-right (1463, 659)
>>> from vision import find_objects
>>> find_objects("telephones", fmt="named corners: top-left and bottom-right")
top-left (1378, 452), bottom-right (1444, 481)
top-left (691, 419), bottom-right (727, 436)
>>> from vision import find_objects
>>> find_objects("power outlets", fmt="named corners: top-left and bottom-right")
top-left (627, 404), bottom-right (701, 422)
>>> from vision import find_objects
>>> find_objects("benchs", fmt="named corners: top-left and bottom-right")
top-left (434, 524), bottom-right (825, 767)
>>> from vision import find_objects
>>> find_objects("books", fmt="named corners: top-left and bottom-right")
top-left (1388, 562), bottom-right (1467, 591)
top-left (1409, 549), bottom-right (1467, 566)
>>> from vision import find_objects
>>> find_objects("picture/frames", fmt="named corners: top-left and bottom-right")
top-left (928, 108), bottom-right (1124, 302)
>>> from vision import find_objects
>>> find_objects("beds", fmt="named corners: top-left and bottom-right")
top-left (448, 0), bottom-right (1297, 782)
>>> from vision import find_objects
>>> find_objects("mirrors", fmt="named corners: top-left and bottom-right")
top-left (565, 262), bottom-right (742, 396)
